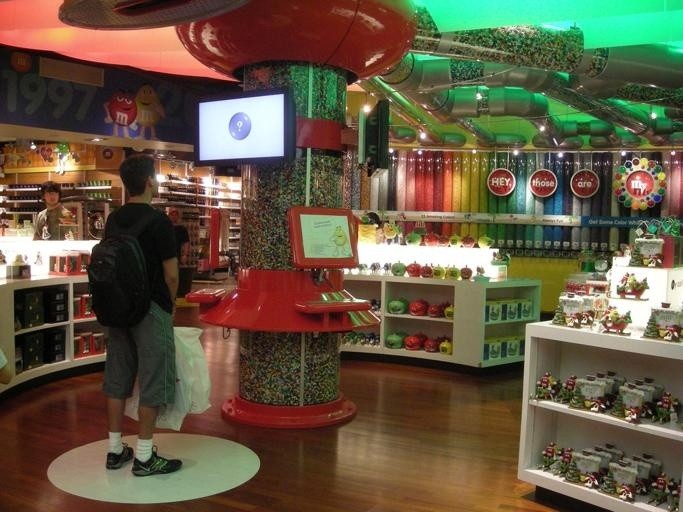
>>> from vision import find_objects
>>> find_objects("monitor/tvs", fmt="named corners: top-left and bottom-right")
top-left (214, 165), bottom-right (241, 177)
top-left (365, 100), bottom-right (389, 179)
top-left (192, 86), bottom-right (296, 168)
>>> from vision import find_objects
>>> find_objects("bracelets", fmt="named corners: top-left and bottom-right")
top-left (185, 253), bottom-right (189, 257)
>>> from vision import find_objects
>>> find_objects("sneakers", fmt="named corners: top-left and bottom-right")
top-left (133, 452), bottom-right (182, 477)
top-left (105, 446), bottom-right (133, 470)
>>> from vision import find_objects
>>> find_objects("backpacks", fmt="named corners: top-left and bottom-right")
top-left (87, 210), bottom-right (162, 328)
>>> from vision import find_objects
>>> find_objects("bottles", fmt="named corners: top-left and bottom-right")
top-left (386, 330), bottom-right (452, 354)
top-left (391, 260), bottom-right (472, 280)
top-left (404, 229), bottom-right (493, 249)
top-left (388, 297), bottom-right (454, 320)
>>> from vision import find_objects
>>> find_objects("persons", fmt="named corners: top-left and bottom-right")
top-left (100, 155), bottom-right (182, 476)
top-left (32, 181), bottom-right (77, 241)
top-left (165, 207), bottom-right (191, 324)
top-left (0, 346), bottom-right (13, 385)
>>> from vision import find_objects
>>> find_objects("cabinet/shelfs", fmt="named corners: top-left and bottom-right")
top-left (340, 273), bottom-right (542, 369)
top-left (0, 179), bottom-right (110, 234)
top-left (0, 269), bottom-right (110, 394)
top-left (518, 317), bottom-right (682, 512)
top-left (160, 177), bottom-right (242, 274)
